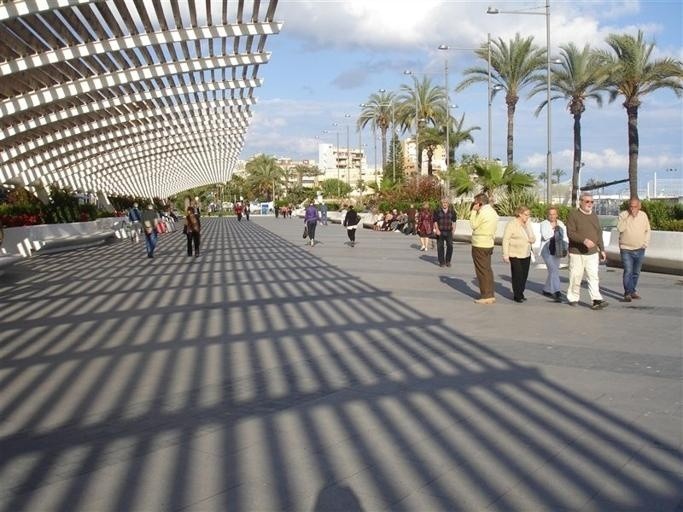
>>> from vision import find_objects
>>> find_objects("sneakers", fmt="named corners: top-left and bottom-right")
top-left (591, 300), bottom-right (609, 310)
top-left (555, 293), bottom-right (569, 304)
top-left (474, 297), bottom-right (495, 305)
top-left (623, 293), bottom-right (632, 302)
top-left (631, 291), bottom-right (640, 298)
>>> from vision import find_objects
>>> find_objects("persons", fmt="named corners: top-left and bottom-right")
top-left (373, 193), bottom-right (498, 304)
top-left (502, 207), bottom-right (536, 302)
top-left (118, 196), bottom-right (250, 258)
top-left (339, 205), bottom-right (348, 225)
top-left (539, 207), bottom-right (569, 302)
top-left (344, 206), bottom-right (357, 244)
top-left (304, 199), bottom-right (320, 247)
top-left (274, 201), bottom-right (294, 218)
top-left (617, 196), bottom-right (650, 301)
top-left (566, 193), bottom-right (608, 310)
top-left (320, 202), bottom-right (327, 225)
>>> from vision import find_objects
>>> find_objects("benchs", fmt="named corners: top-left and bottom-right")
top-left (292, 208), bottom-right (515, 245)
top-left (30, 223), bottom-right (115, 253)
top-left (603, 228), bottom-right (683, 274)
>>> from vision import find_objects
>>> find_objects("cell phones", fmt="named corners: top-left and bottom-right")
top-left (479, 202), bottom-right (483, 207)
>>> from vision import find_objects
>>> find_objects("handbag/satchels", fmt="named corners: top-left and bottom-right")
top-left (303, 227), bottom-right (307, 238)
top-left (182, 223), bottom-right (187, 234)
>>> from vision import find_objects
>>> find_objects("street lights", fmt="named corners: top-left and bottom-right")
top-left (378, 78), bottom-right (426, 189)
top-left (486, 1), bottom-right (564, 206)
top-left (437, 33), bottom-right (503, 165)
top-left (314, 93), bottom-right (401, 198)
top-left (403, 60), bottom-right (458, 197)
